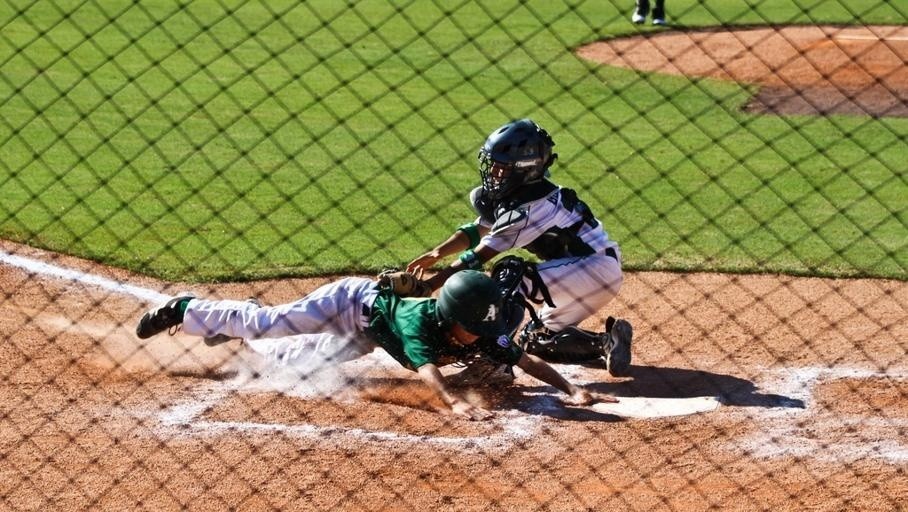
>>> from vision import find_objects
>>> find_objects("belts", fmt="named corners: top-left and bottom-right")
top-left (361, 284), bottom-right (383, 338)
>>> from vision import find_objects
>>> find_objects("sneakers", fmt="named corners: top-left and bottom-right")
top-left (651, 17), bottom-right (666, 25)
top-left (203, 297), bottom-right (261, 346)
top-left (134, 295), bottom-right (197, 339)
top-left (631, 0), bottom-right (649, 25)
top-left (602, 317), bottom-right (634, 377)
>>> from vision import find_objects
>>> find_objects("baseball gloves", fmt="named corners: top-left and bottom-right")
top-left (377, 264), bottom-right (432, 297)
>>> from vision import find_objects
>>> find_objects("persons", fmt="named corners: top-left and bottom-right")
top-left (630, 0), bottom-right (671, 27)
top-left (374, 118), bottom-right (635, 389)
top-left (135, 266), bottom-right (620, 423)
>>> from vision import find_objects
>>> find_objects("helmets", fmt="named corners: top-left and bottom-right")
top-left (433, 268), bottom-right (511, 337)
top-left (474, 118), bottom-right (558, 210)
